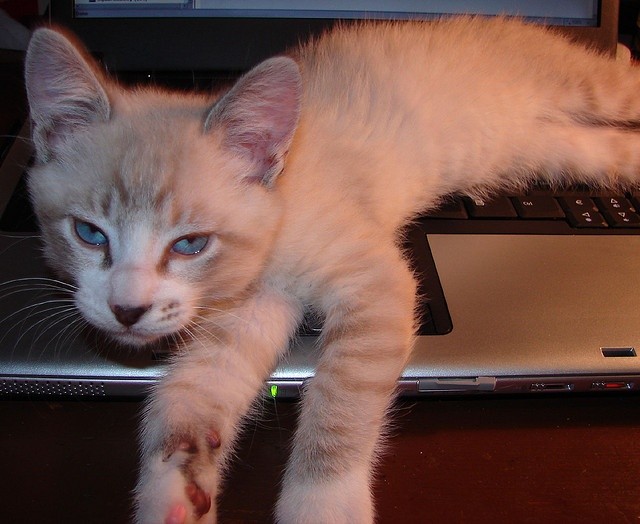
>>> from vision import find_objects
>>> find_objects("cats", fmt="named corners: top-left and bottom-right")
top-left (0, 12), bottom-right (640, 524)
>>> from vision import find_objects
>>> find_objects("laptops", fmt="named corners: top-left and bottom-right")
top-left (0, 0), bottom-right (640, 399)
